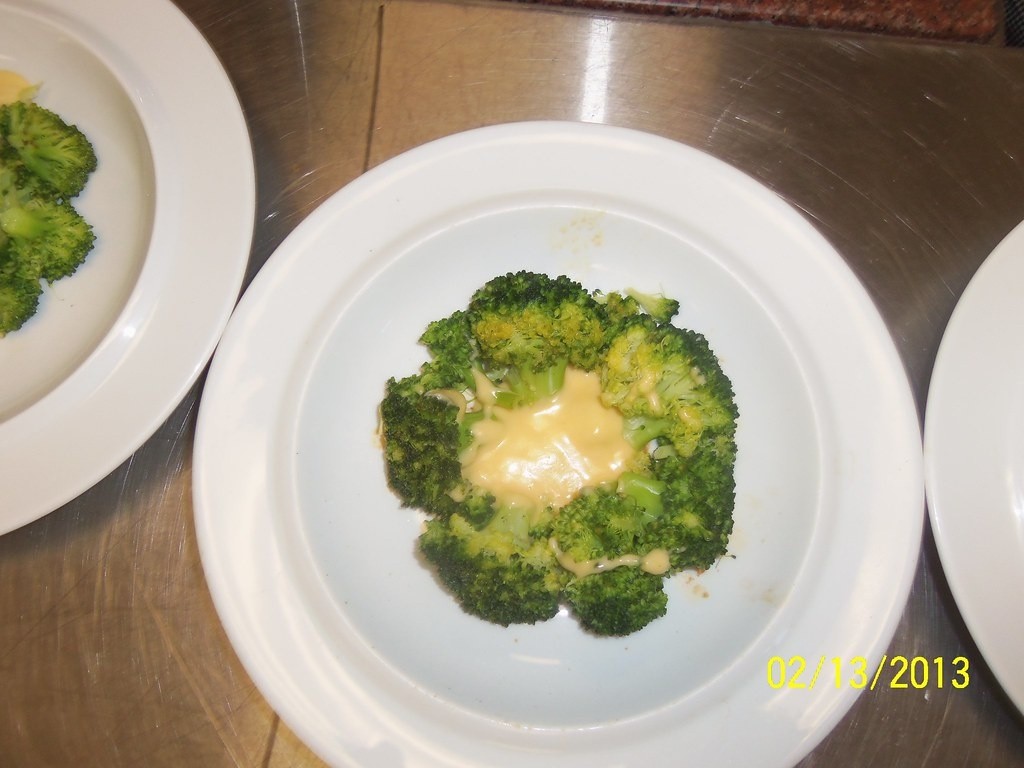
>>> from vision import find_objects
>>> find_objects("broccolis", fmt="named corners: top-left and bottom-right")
top-left (380, 269), bottom-right (740, 638)
top-left (0, 102), bottom-right (101, 338)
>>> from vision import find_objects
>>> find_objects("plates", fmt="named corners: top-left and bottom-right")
top-left (192, 119), bottom-right (924, 768)
top-left (923, 218), bottom-right (1024, 716)
top-left (0, 0), bottom-right (256, 537)
